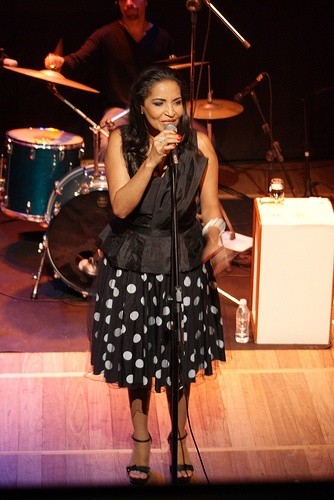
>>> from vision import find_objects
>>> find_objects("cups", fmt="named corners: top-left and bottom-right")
top-left (269, 178), bottom-right (285, 204)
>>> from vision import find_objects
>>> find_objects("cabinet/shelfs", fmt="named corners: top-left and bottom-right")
top-left (249, 198), bottom-right (334, 345)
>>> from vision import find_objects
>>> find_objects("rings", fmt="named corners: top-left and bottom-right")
top-left (159, 137), bottom-right (164, 143)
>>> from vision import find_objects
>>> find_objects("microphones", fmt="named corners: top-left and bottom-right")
top-left (164, 124), bottom-right (178, 165)
top-left (232, 73), bottom-right (264, 103)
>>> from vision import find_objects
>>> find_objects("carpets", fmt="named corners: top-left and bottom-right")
top-left (0, 198), bottom-right (332, 354)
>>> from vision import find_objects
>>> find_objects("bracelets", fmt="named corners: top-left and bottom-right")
top-left (203, 219), bottom-right (224, 236)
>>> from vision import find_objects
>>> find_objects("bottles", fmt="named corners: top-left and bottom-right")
top-left (235, 299), bottom-right (250, 344)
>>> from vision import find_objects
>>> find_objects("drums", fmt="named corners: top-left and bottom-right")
top-left (1, 127), bottom-right (85, 223)
top-left (44, 163), bottom-right (124, 296)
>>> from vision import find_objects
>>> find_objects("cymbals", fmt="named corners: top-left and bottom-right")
top-left (185, 98), bottom-right (244, 120)
top-left (168, 61), bottom-right (210, 69)
top-left (4, 64), bottom-right (100, 94)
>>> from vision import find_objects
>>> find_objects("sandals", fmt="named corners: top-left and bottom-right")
top-left (126, 431), bottom-right (153, 483)
top-left (167, 429), bottom-right (194, 482)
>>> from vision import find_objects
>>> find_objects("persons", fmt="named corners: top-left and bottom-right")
top-left (90, 64), bottom-right (226, 483)
top-left (46, 0), bottom-right (175, 162)
